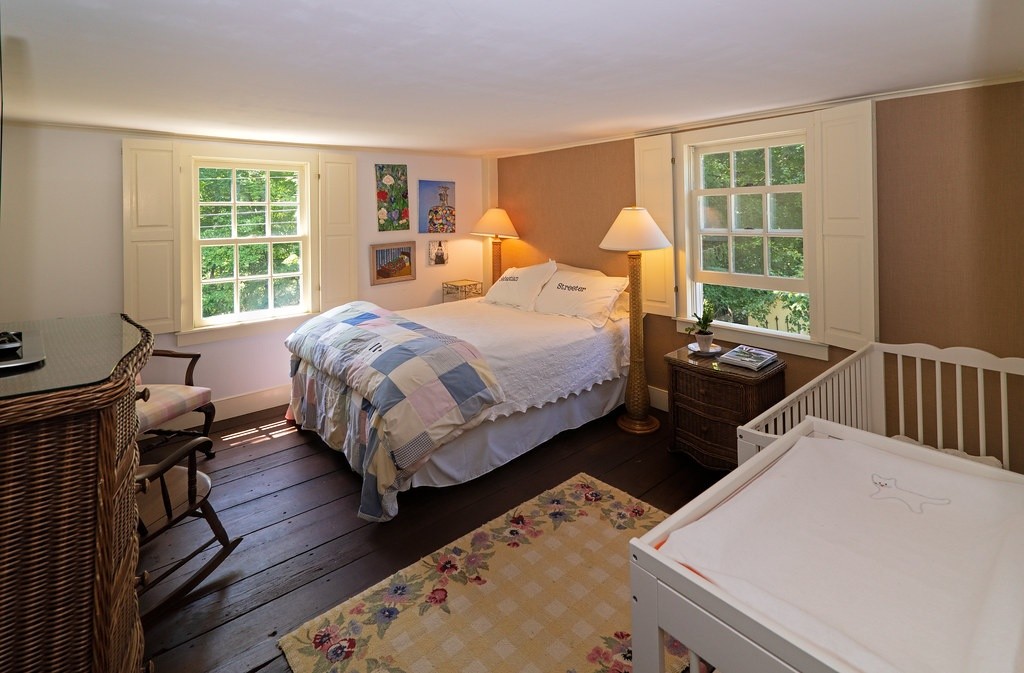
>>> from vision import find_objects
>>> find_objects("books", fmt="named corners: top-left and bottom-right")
top-left (722, 343), bottom-right (779, 370)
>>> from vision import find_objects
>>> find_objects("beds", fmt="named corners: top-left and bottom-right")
top-left (284, 260), bottom-right (634, 524)
top-left (737, 343), bottom-right (1024, 471)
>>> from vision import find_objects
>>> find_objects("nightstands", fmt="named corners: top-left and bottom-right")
top-left (666, 341), bottom-right (786, 469)
top-left (443, 279), bottom-right (483, 303)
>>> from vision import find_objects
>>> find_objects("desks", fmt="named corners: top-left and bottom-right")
top-left (629, 416), bottom-right (1024, 673)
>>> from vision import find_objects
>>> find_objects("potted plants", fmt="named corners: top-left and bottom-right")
top-left (685, 303), bottom-right (716, 351)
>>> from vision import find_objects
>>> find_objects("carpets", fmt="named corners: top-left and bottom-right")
top-left (276, 472), bottom-right (704, 673)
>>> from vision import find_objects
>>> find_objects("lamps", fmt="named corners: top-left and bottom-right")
top-left (469, 208), bottom-right (520, 284)
top-left (597, 207), bottom-right (673, 434)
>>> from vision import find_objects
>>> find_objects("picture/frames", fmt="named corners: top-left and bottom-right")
top-left (368, 241), bottom-right (417, 286)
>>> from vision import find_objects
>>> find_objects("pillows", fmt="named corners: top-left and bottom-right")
top-left (482, 260), bottom-right (558, 309)
top-left (534, 269), bottom-right (630, 329)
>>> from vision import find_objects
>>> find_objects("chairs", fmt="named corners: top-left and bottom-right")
top-left (135, 323), bottom-right (246, 624)
